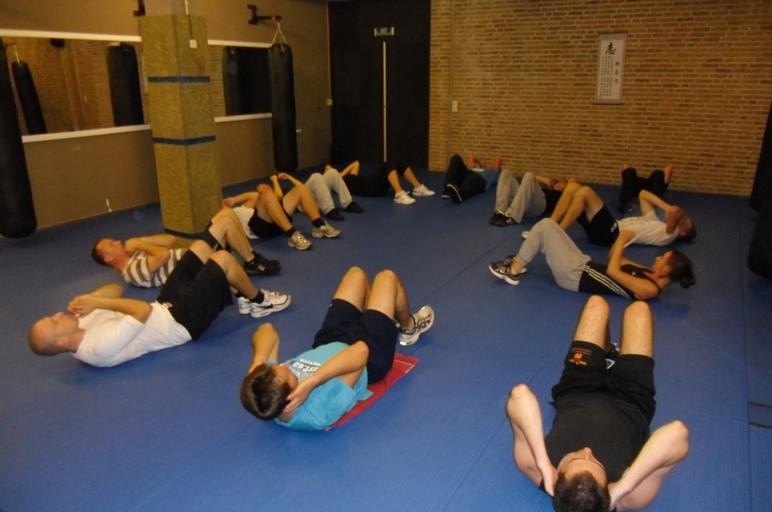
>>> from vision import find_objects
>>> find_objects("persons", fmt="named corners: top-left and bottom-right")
top-left (488, 167), bottom-right (578, 226)
top-left (208, 183), bottom-right (343, 251)
top-left (255, 169), bottom-right (366, 222)
top-left (90, 206), bottom-right (282, 290)
top-left (239, 264), bottom-right (435, 431)
top-left (519, 182), bottom-right (699, 247)
top-left (487, 216), bottom-right (697, 302)
top-left (504, 294), bottom-right (690, 512)
top-left (440, 152), bottom-right (503, 203)
top-left (615, 162), bottom-right (674, 215)
top-left (27, 238), bottom-right (293, 369)
top-left (318, 155), bottom-right (436, 206)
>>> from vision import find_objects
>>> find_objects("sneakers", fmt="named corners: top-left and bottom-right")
top-left (324, 209), bottom-right (344, 221)
top-left (250, 289), bottom-right (292, 318)
top-left (413, 184), bottom-right (435, 196)
top-left (495, 215), bottom-right (511, 226)
top-left (398, 305), bottom-right (435, 347)
top-left (488, 212), bottom-right (500, 224)
top-left (394, 190), bottom-right (416, 205)
top-left (244, 260), bottom-right (281, 277)
top-left (446, 184), bottom-right (463, 203)
top-left (237, 291), bottom-right (279, 315)
top-left (287, 230), bottom-right (312, 250)
top-left (311, 220), bottom-right (341, 239)
top-left (258, 254), bottom-right (279, 264)
top-left (345, 202), bottom-right (365, 213)
top-left (488, 262), bottom-right (521, 286)
top-left (441, 189), bottom-right (450, 199)
